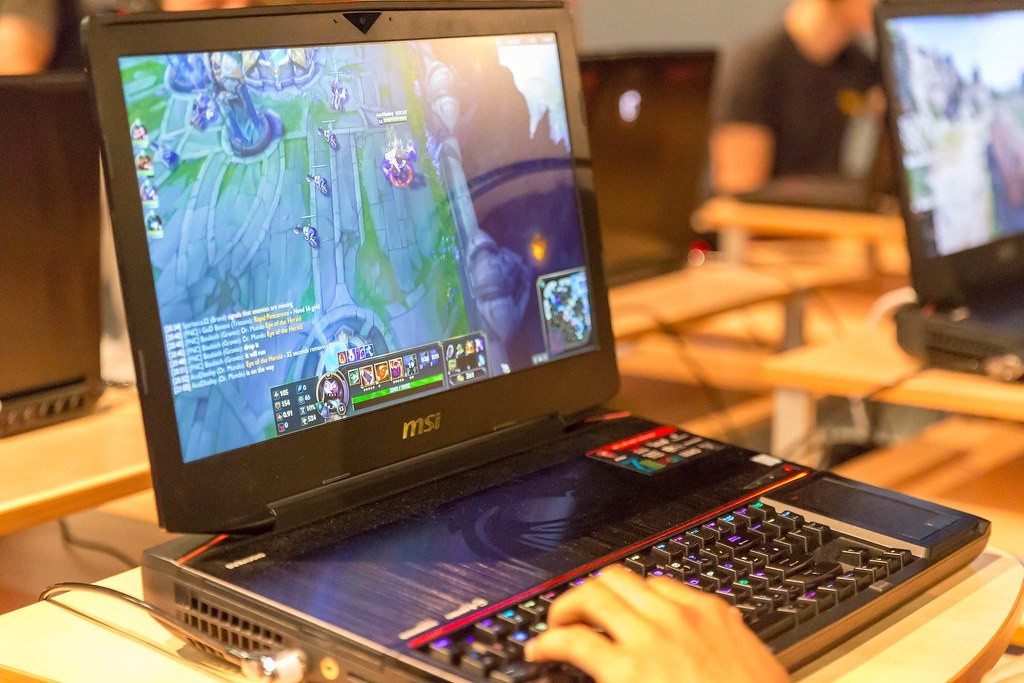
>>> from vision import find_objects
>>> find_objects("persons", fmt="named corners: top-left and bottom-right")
top-left (522, 562), bottom-right (793, 682)
top-left (702, 1), bottom-right (885, 210)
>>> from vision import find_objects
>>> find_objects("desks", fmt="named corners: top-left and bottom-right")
top-left (0, 384), bottom-right (153, 539)
top-left (609, 263), bottom-right (842, 350)
top-left (761, 325), bottom-right (1023, 500)
top-left (0, 543), bottom-right (1024, 682)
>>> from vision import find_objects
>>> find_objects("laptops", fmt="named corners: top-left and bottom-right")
top-left (0, 0), bottom-right (1024, 683)
